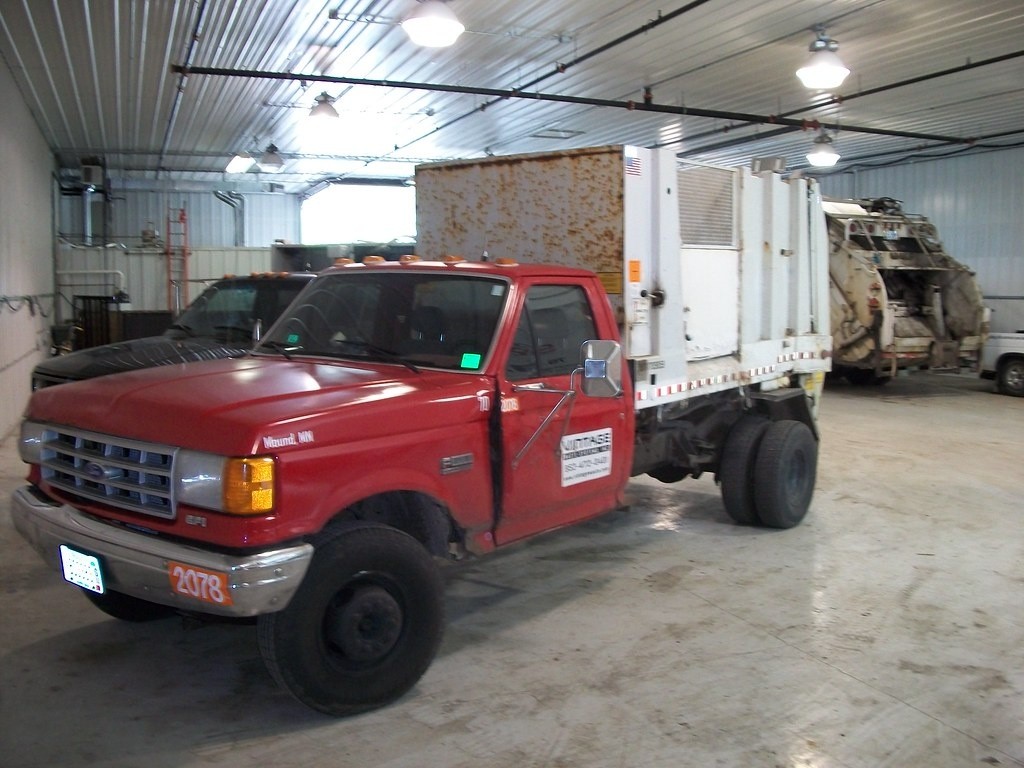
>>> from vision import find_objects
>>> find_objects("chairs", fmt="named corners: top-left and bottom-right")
top-left (394, 305), bottom-right (450, 356)
top-left (519, 306), bottom-right (572, 374)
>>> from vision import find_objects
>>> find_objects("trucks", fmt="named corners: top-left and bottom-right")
top-left (978, 333), bottom-right (1024, 397)
top-left (10, 145), bottom-right (833, 717)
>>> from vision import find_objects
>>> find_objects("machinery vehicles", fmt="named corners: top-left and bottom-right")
top-left (820, 195), bottom-right (990, 383)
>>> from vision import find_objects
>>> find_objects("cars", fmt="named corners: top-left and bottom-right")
top-left (31, 271), bottom-right (317, 394)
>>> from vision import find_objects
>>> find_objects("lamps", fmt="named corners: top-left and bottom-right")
top-left (397, 0), bottom-right (465, 51)
top-left (309, 91), bottom-right (340, 118)
top-left (792, 27), bottom-right (851, 94)
top-left (224, 152), bottom-right (256, 173)
top-left (256, 143), bottom-right (285, 173)
top-left (805, 124), bottom-right (842, 167)
